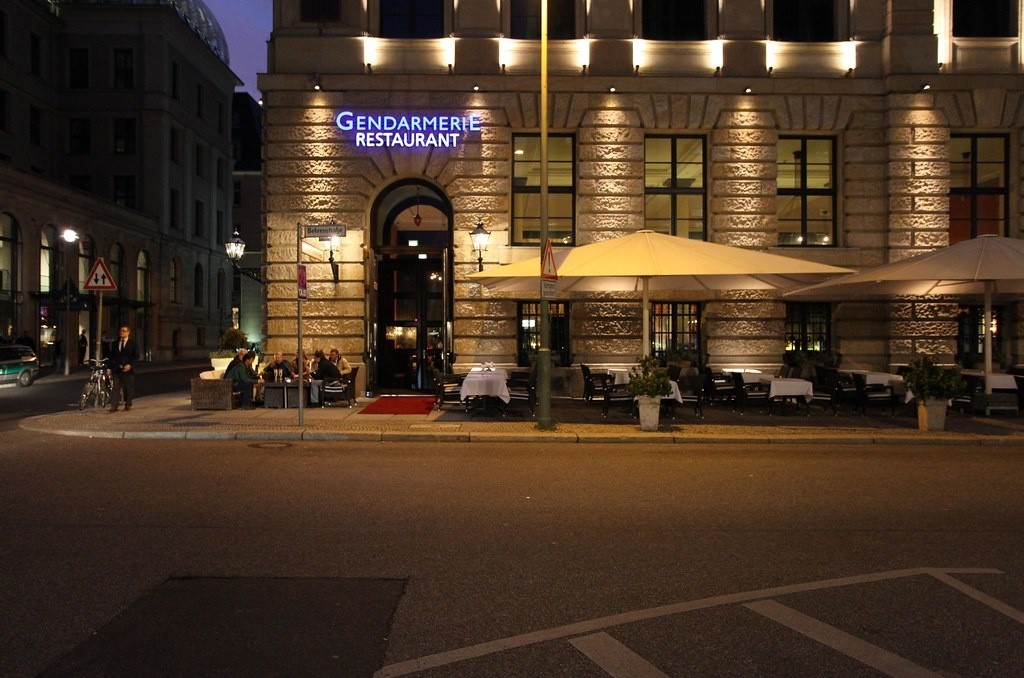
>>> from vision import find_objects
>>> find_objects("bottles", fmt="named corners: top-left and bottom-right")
top-left (274, 369), bottom-right (278, 381)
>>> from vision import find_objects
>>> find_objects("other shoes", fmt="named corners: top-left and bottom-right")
top-left (124, 406), bottom-right (131, 411)
top-left (108, 407), bottom-right (117, 413)
top-left (242, 403), bottom-right (255, 409)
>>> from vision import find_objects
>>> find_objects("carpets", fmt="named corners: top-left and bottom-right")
top-left (356, 396), bottom-right (437, 414)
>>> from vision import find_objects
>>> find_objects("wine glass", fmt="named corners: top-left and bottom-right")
top-left (279, 369), bottom-right (283, 381)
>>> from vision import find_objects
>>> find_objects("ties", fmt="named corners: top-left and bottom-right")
top-left (121, 340), bottom-right (124, 351)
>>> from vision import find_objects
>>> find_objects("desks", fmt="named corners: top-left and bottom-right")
top-left (460, 366), bottom-right (510, 417)
top-left (852, 371), bottom-right (903, 418)
top-left (836, 369), bottom-right (867, 390)
top-left (962, 369), bottom-right (1017, 415)
top-left (722, 367), bottom-right (762, 393)
top-left (606, 368), bottom-right (651, 409)
top-left (768, 377), bottom-right (813, 417)
top-left (259, 381), bottom-right (311, 408)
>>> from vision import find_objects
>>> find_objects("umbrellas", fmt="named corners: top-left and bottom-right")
top-left (783, 233), bottom-right (1024, 393)
top-left (466, 229), bottom-right (857, 360)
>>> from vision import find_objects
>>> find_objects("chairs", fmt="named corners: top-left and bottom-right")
top-left (579, 363), bottom-right (986, 422)
top-left (434, 369), bottom-right (467, 412)
top-left (321, 366), bottom-right (360, 409)
top-left (508, 362), bottom-right (538, 417)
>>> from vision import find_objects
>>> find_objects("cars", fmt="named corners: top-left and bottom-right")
top-left (0, 344), bottom-right (41, 388)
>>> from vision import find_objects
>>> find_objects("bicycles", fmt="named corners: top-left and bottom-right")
top-left (79, 356), bottom-right (125, 412)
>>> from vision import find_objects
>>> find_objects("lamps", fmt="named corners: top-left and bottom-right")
top-left (469, 217), bottom-right (491, 271)
top-left (324, 217), bottom-right (339, 282)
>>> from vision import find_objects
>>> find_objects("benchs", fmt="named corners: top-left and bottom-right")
top-left (190, 375), bottom-right (242, 411)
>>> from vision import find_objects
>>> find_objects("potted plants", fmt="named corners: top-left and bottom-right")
top-left (626, 363), bottom-right (674, 431)
top-left (905, 350), bottom-right (969, 430)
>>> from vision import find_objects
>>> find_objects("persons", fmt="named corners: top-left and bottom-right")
top-left (54, 334), bottom-right (64, 358)
top-left (78, 329), bottom-right (87, 366)
top-left (306, 350), bottom-right (341, 408)
top-left (291, 349), bottom-right (309, 379)
top-left (262, 352), bottom-right (292, 382)
top-left (106, 323), bottom-right (137, 412)
top-left (328, 349), bottom-right (352, 376)
top-left (22, 329), bottom-right (33, 348)
top-left (223, 348), bottom-right (265, 410)
top-left (101, 331), bottom-right (110, 358)
